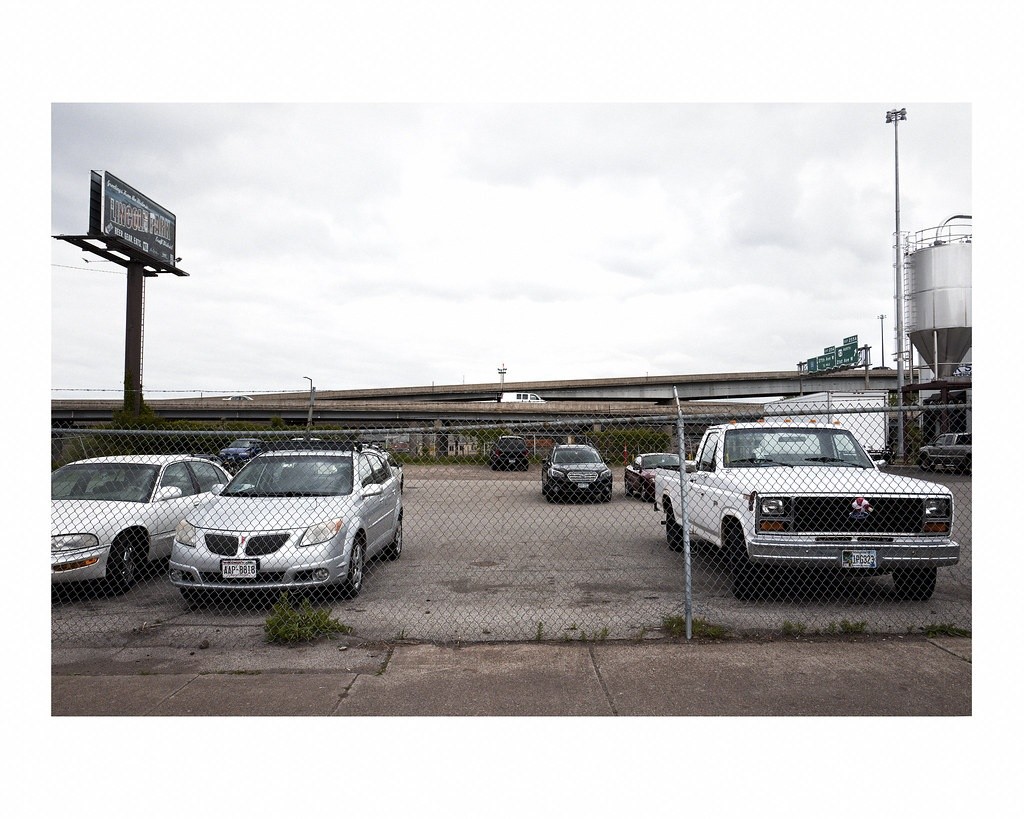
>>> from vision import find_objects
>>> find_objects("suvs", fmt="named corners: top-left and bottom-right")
top-left (916, 432), bottom-right (972, 475)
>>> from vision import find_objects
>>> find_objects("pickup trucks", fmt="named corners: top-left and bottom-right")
top-left (653, 419), bottom-right (960, 602)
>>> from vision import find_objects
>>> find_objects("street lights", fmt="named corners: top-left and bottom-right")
top-left (885, 106), bottom-right (910, 466)
top-left (302, 376), bottom-right (316, 431)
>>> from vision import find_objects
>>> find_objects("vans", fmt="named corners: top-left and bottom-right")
top-left (500, 391), bottom-right (547, 403)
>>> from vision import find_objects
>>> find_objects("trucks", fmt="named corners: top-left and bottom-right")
top-left (749, 389), bottom-right (889, 464)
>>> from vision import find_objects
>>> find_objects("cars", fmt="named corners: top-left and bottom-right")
top-left (272, 442), bottom-right (406, 493)
top-left (541, 441), bottom-right (612, 501)
top-left (180, 453), bottom-right (235, 476)
top-left (168, 440), bottom-right (405, 602)
top-left (51, 455), bottom-right (235, 595)
top-left (489, 435), bottom-right (530, 470)
top-left (217, 437), bottom-right (263, 465)
top-left (221, 395), bottom-right (255, 401)
top-left (624, 453), bottom-right (679, 502)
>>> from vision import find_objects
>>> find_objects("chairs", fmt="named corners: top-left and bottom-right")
top-left (91, 474), bottom-right (215, 495)
top-left (267, 462), bottom-right (353, 495)
